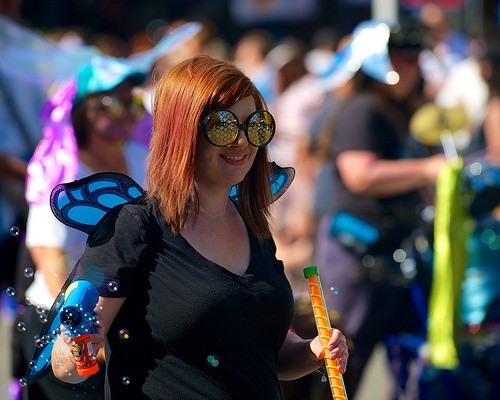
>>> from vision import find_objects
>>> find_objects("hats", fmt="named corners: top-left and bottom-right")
top-left (73, 53), bottom-right (147, 102)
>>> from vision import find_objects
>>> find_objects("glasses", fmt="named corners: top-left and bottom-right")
top-left (197, 109), bottom-right (276, 147)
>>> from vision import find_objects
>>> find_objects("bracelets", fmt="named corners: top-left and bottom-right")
top-left (306, 338), bottom-right (327, 382)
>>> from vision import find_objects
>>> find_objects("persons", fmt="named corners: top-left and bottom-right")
top-left (53, 57), bottom-right (352, 400)
top-left (0, 1), bottom-right (499, 400)
